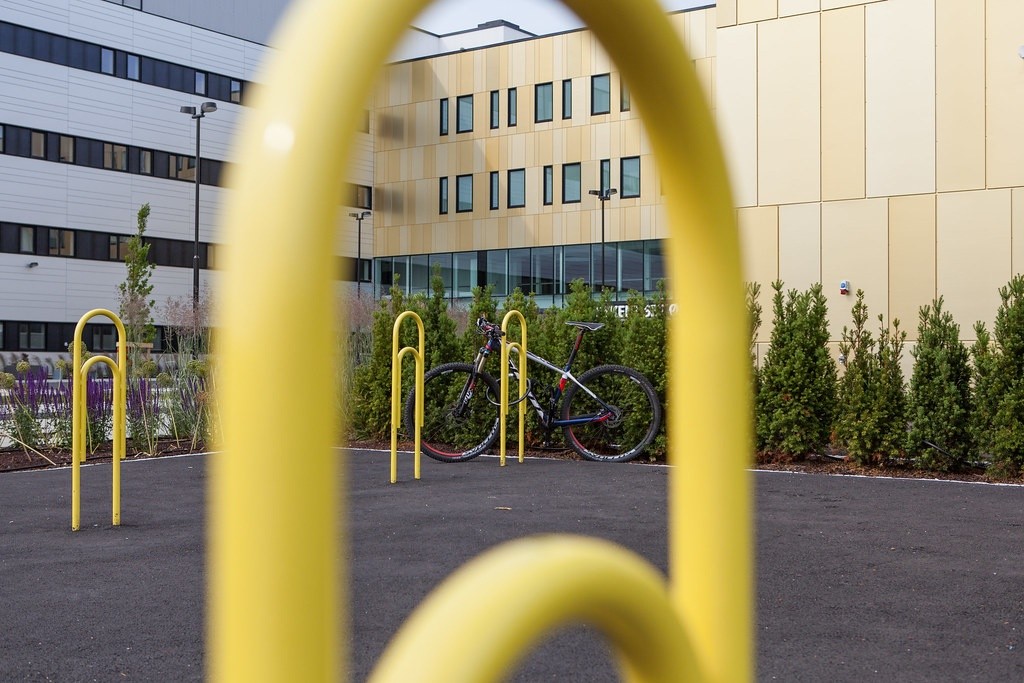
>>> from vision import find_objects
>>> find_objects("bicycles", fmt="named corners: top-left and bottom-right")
top-left (404, 313), bottom-right (662, 463)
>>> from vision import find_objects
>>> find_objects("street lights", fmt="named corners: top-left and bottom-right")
top-left (180, 101), bottom-right (218, 358)
top-left (348, 211), bottom-right (372, 365)
top-left (588, 187), bottom-right (617, 286)
